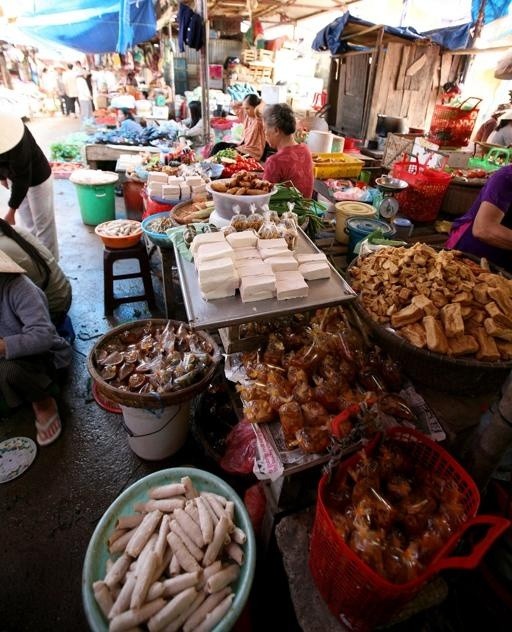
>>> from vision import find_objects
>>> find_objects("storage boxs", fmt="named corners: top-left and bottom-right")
top-left (241, 48), bottom-right (274, 64)
top-left (248, 65), bottom-right (272, 78)
top-left (407, 138), bottom-right (471, 175)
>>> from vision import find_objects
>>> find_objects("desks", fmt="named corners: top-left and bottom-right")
top-left (142, 197), bottom-right (179, 320)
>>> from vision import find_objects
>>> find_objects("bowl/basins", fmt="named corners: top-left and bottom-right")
top-left (141, 211), bottom-right (175, 249)
top-left (95, 220), bottom-right (144, 250)
top-left (205, 178), bottom-right (278, 220)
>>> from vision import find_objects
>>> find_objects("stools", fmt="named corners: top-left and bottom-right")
top-left (102, 243), bottom-right (154, 316)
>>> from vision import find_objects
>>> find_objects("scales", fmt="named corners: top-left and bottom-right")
top-left (374, 177), bottom-right (408, 220)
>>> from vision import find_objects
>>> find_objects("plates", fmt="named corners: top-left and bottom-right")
top-left (0, 436), bottom-right (37, 484)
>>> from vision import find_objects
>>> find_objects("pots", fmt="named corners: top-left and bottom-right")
top-left (374, 112), bottom-right (410, 138)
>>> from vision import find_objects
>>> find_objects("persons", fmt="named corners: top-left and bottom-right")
top-left (263, 101), bottom-right (314, 199)
top-left (441, 163), bottom-right (512, 275)
top-left (211, 94), bottom-right (266, 161)
top-left (0, 218), bottom-right (72, 321)
top-left (118, 107), bottom-right (141, 135)
top-left (473, 103), bottom-right (512, 158)
top-left (0, 250), bottom-right (73, 445)
top-left (183, 99), bottom-right (215, 146)
top-left (41, 60), bottom-right (96, 114)
top-left (0, 106), bottom-right (59, 261)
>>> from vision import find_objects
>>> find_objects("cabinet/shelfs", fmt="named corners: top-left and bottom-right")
top-left (86, 144), bottom-right (159, 169)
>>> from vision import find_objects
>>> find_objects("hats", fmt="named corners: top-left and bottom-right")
top-left (0, 110), bottom-right (25, 154)
top-left (0, 249), bottom-right (26, 273)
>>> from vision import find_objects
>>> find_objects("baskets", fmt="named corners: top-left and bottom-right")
top-left (428, 94), bottom-right (482, 146)
top-left (311, 153), bottom-right (365, 179)
top-left (306, 405), bottom-right (511, 630)
top-left (394, 151), bottom-right (455, 222)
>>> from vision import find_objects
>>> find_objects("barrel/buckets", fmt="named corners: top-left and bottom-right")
top-left (334, 201), bottom-right (377, 245)
top-left (119, 401), bottom-right (191, 461)
top-left (392, 217), bottom-right (411, 241)
top-left (122, 180), bottom-right (144, 220)
top-left (75, 183), bottom-right (115, 227)
top-left (360, 171), bottom-right (371, 182)
top-left (344, 217), bottom-right (395, 265)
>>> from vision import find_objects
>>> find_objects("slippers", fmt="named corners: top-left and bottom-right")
top-left (35, 399), bottom-right (62, 446)
